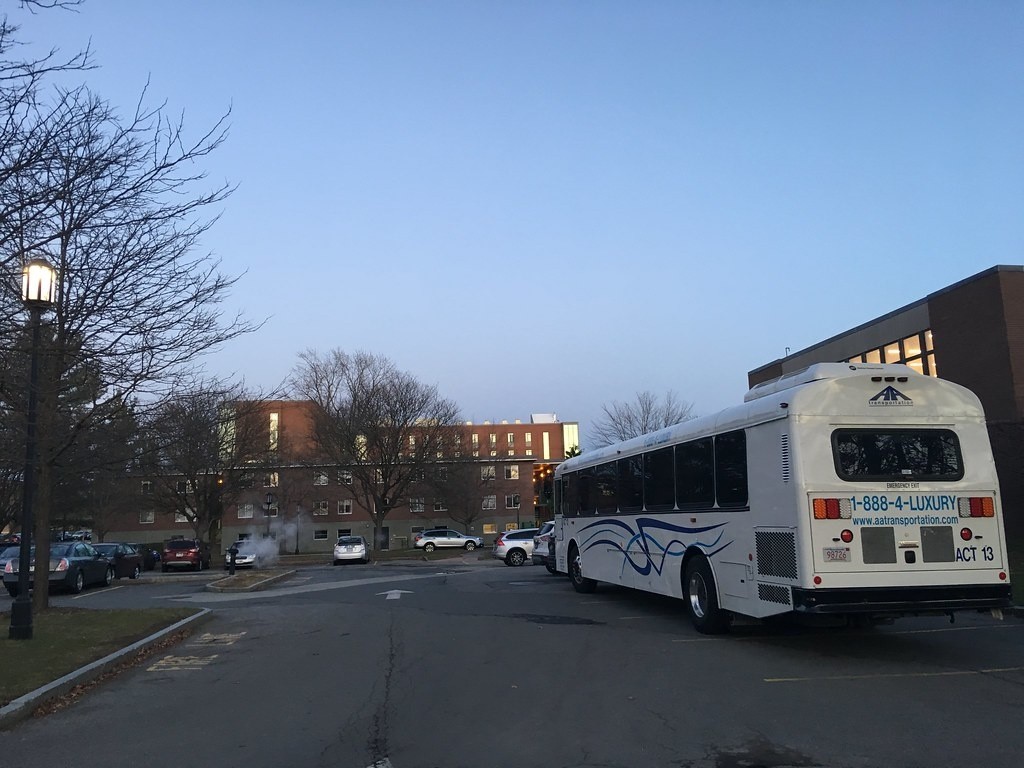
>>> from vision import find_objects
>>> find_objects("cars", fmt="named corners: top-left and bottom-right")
top-left (2, 541), bottom-right (112, 597)
top-left (91, 542), bottom-right (144, 579)
top-left (0, 529), bottom-right (92, 544)
top-left (531, 520), bottom-right (555, 573)
top-left (153, 550), bottom-right (160, 561)
top-left (225, 539), bottom-right (265, 570)
top-left (493, 527), bottom-right (540, 566)
top-left (0, 545), bottom-right (20, 577)
top-left (333, 535), bottom-right (372, 564)
top-left (126, 542), bottom-right (156, 571)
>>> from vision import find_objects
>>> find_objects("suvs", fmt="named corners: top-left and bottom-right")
top-left (414, 527), bottom-right (485, 553)
top-left (160, 534), bottom-right (212, 571)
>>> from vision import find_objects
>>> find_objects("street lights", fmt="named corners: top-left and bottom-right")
top-left (295, 504), bottom-right (301, 555)
top-left (9, 256), bottom-right (60, 641)
top-left (514, 494), bottom-right (521, 530)
top-left (266, 493), bottom-right (273, 536)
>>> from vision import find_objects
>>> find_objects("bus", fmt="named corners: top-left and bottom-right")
top-left (548, 360), bottom-right (1012, 636)
